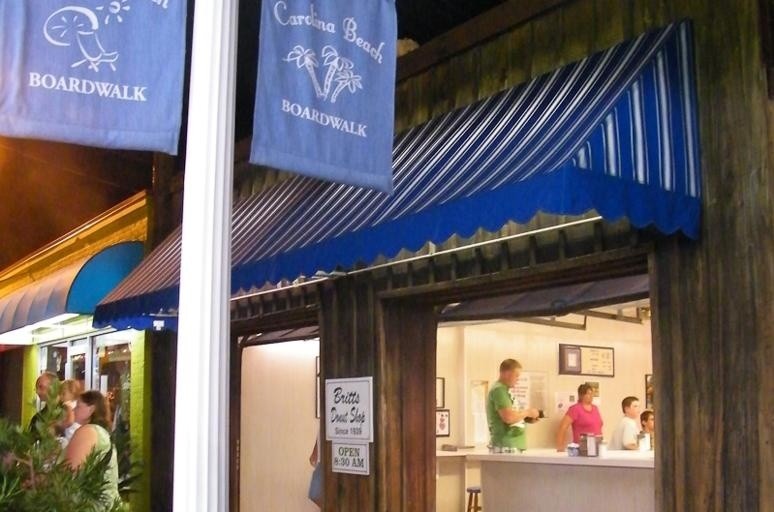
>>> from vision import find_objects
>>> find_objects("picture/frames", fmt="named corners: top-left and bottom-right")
top-left (435, 375), bottom-right (450, 438)
top-left (644, 373), bottom-right (654, 409)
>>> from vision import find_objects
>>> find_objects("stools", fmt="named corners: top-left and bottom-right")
top-left (466, 486), bottom-right (482, 512)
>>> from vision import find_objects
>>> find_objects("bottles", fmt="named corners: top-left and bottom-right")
top-left (567, 443), bottom-right (579, 456)
top-left (579, 432), bottom-right (599, 457)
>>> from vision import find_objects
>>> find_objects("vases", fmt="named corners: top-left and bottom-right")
top-left (558, 343), bottom-right (614, 378)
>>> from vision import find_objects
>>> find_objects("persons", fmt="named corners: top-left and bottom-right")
top-left (554, 383), bottom-right (602, 454)
top-left (37, 378), bottom-right (82, 450)
top-left (635, 410), bottom-right (655, 453)
top-left (485, 357), bottom-right (542, 454)
top-left (25, 371), bottom-right (63, 470)
top-left (21, 388), bottom-right (119, 510)
top-left (609, 395), bottom-right (645, 451)
